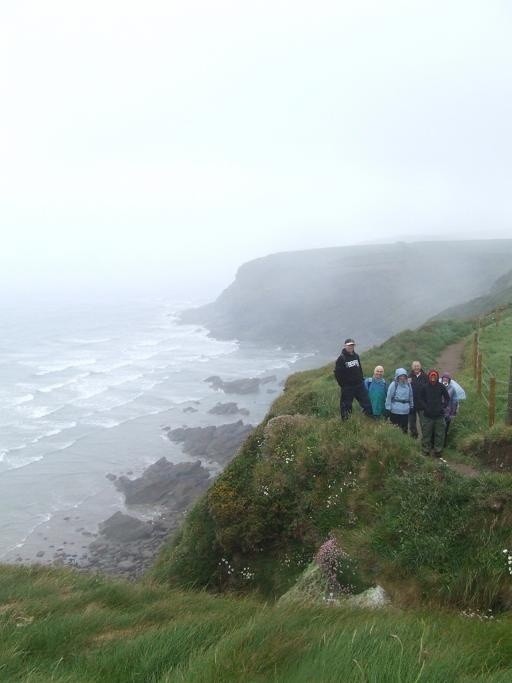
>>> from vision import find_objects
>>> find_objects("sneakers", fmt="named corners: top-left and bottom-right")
top-left (422, 450), bottom-right (442, 458)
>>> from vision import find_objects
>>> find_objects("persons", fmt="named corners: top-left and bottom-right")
top-left (384, 367), bottom-right (414, 434)
top-left (439, 372), bottom-right (466, 447)
top-left (361, 365), bottom-right (389, 421)
top-left (407, 359), bottom-right (428, 439)
top-left (417, 369), bottom-right (450, 458)
top-left (333, 337), bottom-right (374, 421)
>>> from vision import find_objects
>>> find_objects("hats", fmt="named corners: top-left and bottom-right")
top-left (345, 339), bottom-right (356, 346)
